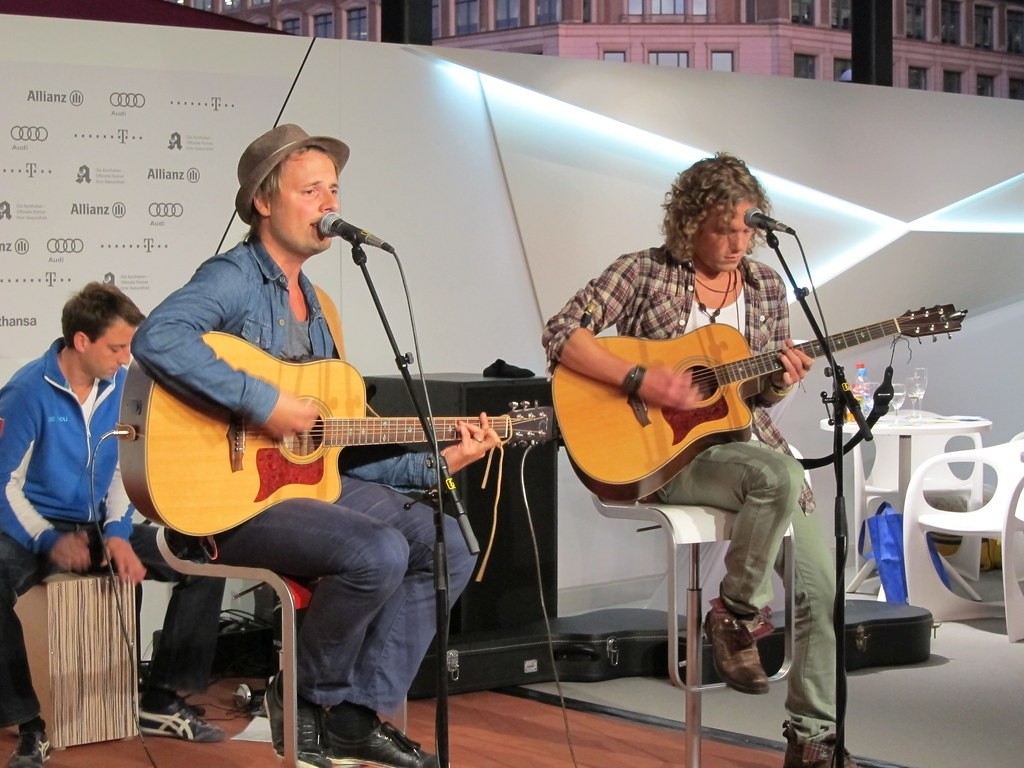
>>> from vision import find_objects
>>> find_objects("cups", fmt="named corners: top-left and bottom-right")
top-left (862, 382), bottom-right (881, 425)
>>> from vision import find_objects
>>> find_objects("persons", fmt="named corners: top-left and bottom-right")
top-left (541, 156), bottom-right (859, 768)
top-left (129, 124), bottom-right (501, 768)
top-left (0, 283), bottom-right (228, 768)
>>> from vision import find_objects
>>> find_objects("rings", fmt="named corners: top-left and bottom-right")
top-left (473, 438), bottom-right (482, 443)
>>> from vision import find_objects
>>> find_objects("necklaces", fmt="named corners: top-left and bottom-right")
top-left (695, 270), bottom-right (737, 293)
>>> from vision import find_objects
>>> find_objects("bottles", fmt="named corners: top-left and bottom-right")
top-left (854, 363), bottom-right (872, 420)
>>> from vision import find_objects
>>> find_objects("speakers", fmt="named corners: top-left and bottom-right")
top-left (341, 372), bottom-right (559, 633)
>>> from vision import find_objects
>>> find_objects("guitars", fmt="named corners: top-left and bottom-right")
top-left (550, 302), bottom-right (969, 503)
top-left (120, 333), bottom-right (550, 536)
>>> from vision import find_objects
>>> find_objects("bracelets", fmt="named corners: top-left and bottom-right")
top-left (620, 366), bottom-right (646, 393)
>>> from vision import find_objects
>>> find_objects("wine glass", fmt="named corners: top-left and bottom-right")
top-left (889, 383), bottom-right (907, 428)
top-left (905, 376), bottom-right (922, 420)
top-left (913, 368), bottom-right (930, 424)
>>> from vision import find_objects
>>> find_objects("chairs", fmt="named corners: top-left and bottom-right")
top-left (898, 432), bottom-right (1024, 644)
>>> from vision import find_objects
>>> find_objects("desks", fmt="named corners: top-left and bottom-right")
top-left (817, 416), bottom-right (990, 605)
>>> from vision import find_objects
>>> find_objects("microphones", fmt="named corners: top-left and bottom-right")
top-left (318, 212), bottom-right (394, 253)
top-left (744, 207), bottom-right (796, 235)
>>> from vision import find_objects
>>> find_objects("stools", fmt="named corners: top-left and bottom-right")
top-left (593, 487), bottom-right (808, 766)
top-left (155, 526), bottom-right (407, 768)
